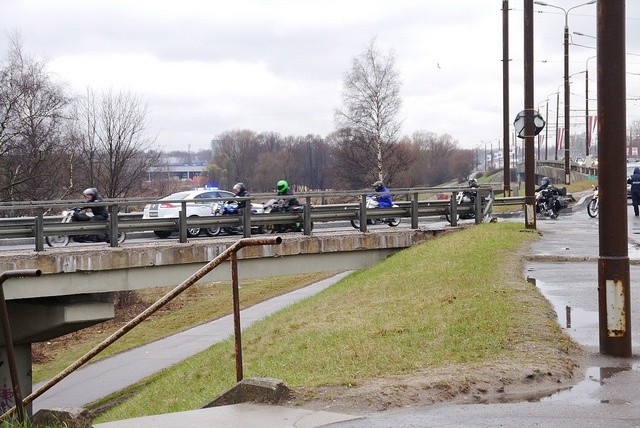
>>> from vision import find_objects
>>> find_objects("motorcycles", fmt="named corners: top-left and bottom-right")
top-left (205, 197), bottom-right (263, 237)
top-left (535, 191), bottom-right (560, 219)
top-left (351, 195), bottom-right (401, 228)
top-left (45, 207), bottom-right (126, 247)
top-left (587, 184), bottom-right (600, 218)
top-left (445, 191), bottom-right (489, 223)
top-left (259, 199), bottom-right (313, 234)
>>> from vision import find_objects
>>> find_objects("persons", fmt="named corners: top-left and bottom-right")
top-left (535, 174), bottom-right (559, 217)
top-left (626, 167), bottom-right (640, 216)
top-left (225, 183), bottom-right (252, 211)
top-left (462, 179), bottom-right (484, 203)
top-left (72, 186), bottom-right (108, 225)
top-left (370, 181), bottom-right (391, 210)
top-left (278, 180), bottom-right (300, 208)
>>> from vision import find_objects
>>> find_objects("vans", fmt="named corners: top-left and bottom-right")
top-left (627, 163), bottom-right (640, 195)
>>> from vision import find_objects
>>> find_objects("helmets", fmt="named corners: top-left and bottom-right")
top-left (82, 188), bottom-right (98, 200)
top-left (541, 177), bottom-right (550, 185)
top-left (469, 178), bottom-right (478, 187)
top-left (371, 181), bottom-right (384, 191)
top-left (233, 183), bottom-right (245, 193)
top-left (277, 180), bottom-right (288, 192)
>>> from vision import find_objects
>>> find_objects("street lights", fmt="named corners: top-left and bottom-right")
top-left (555, 82), bottom-right (573, 160)
top-left (545, 92), bottom-right (561, 160)
top-left (538, 99), bottom-right (551, 160)
top-left (534, 0), bottom-right (596, 185)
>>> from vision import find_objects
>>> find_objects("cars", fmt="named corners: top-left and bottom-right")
top-left (141, 190), bottom-right (264, 238)
